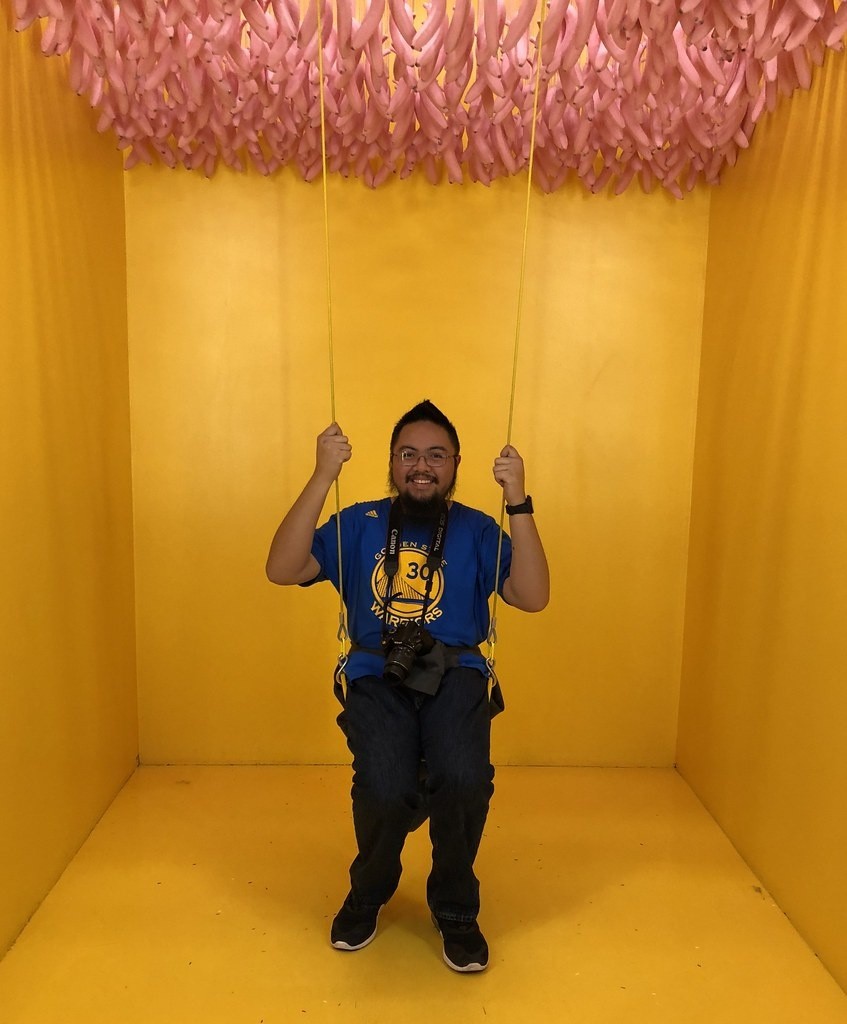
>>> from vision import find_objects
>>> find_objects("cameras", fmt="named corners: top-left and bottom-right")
top-left (381, 620), bottom-right (437, 686)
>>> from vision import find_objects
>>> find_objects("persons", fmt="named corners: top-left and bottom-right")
top-left (263, 400), bottom-right (551, 975)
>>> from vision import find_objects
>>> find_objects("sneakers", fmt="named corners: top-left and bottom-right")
top-left (431, 910), bottom-right (491, 973)
top-left (329, 889), bottom-right (386, 951)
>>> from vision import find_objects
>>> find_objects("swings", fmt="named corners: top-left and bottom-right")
top-left (316, 0), bottom-right (551, 727)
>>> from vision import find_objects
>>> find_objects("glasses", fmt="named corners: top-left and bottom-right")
top-left (391, 449), bottom-right (458, 467)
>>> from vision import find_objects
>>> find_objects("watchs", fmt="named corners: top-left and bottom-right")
top-left (506, 496), bottom-right (536, 515)
